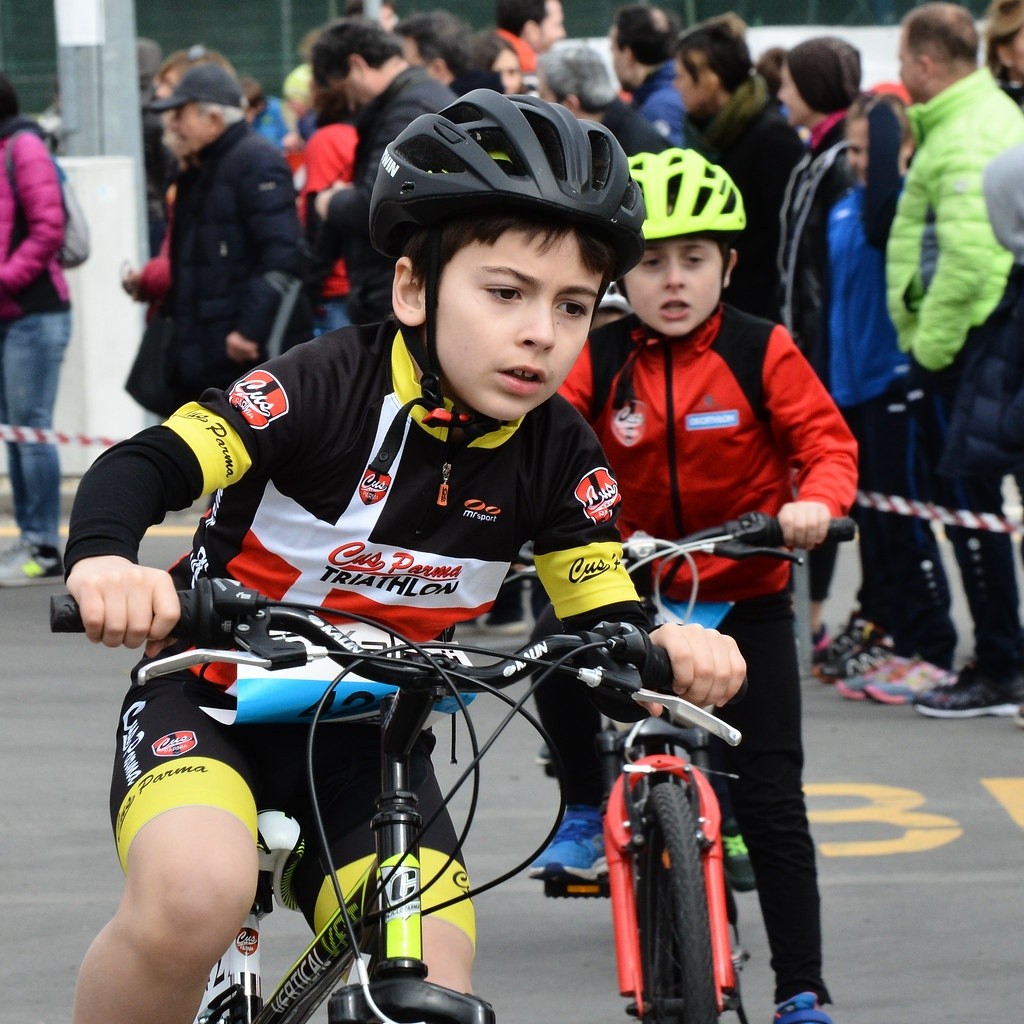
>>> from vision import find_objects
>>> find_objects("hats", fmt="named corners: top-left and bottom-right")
top-left (142, 63), bottom-right (246, 112)
top-left (788, 36), bottom-right (860, 113)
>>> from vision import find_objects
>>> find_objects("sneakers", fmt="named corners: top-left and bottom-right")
top-left (0, 549), bottom-right (64, 585)
top-left (836, 655), bottom-right (918, 700)
top-left (529, 805), bottom-right (608, 882)
top-left (722, 821), bottom-right (756, 889)
top-left (774, 992), bottom-right (831, 1024)
top-left (863, 662), bottom-right (957, 703)
top-left (820, 618), bottom-right (900, 683)
top-left (912, 665), bottom-right (1024, 717)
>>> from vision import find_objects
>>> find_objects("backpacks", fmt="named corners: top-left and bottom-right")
top-left (5, 130), bottom-right (89, 267)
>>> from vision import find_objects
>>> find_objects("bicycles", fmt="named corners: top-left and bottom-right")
top-left (49, 575), bottom-right (740, 1024)
top-left (515, 515), bottom-right (858, 1024)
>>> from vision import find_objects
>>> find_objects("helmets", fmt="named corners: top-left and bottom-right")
top-left (624, 148), bottom-right (746, 241)
top-left (369, 89), bottom-right (647, 278)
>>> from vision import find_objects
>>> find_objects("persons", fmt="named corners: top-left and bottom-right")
top-left (0, 72), bottom-right (74, 587)
top-left (65, 87), bottom-right (742, 1024)
top-left (116, 0), bottom-right (1024, 713)
top-left (527, 145), bottom-right (858, 1024)
